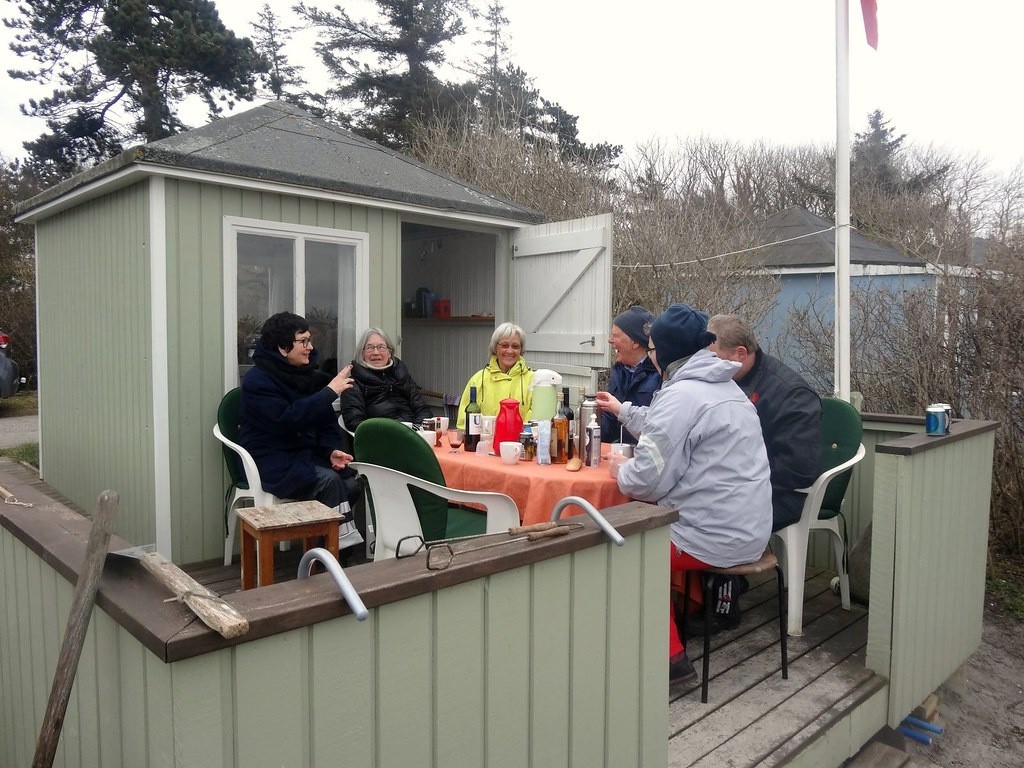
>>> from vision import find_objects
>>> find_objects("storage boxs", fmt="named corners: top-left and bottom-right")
top-left (431, 299), bottom-right (452, 320)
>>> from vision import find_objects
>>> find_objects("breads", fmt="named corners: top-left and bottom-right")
top-left (566, 458), bottom-right (581, 470)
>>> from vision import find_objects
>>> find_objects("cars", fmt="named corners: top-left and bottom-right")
top-left (0, 328), bottom-right (21, 398)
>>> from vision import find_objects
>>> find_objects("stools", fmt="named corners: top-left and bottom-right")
top-left (680, 549), bottom-right (792, 706)
top-left (232, 496), bottom-right (346, 595)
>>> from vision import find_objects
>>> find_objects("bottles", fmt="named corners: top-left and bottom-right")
top-left (434, 417), bottom-right (442, 447)
top-left (584, 414), bottom-right (601, 467)
top-left (463, 387), bottom-right (480, 452)
top-left (559, 387), bottom-right (574, 459)
top-left (550, 392), bottom-right (568, 464)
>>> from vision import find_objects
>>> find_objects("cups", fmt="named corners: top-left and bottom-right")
top-left (405, 287), bottom-right (432, 317)
top-left (532, 385), bottom-right (557, 421)
top-left (401, 422), bottom-right (412, 429)
top-left (448, 429), bottom-right (464, 454)
top-left (500, 441), bottom-right (522, 464)
top-left (432, 417), bottom-right (449, 435)
top-left (574, 435), bottom-right (579, 454)
top-left (475, 416), bottom-right (497, 454)
top-left (421, 430), bottom-right (437, 450)
top-left (611, 443), bottom-right (632, 459)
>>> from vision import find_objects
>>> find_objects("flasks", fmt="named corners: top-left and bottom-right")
top-left (579, 395), bottom-right (601, 462)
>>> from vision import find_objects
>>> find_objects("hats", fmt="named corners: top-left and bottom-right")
top-left (650, 304), bottom-right (717, 371)
top-left (613, 306), bottom-right (654, 347)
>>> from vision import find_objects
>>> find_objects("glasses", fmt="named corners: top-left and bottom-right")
top-left (293, 338), bottom-right (311, 348)
top-left (497, 342), bottom-right (521, 349)
top-left (645, 349), bottom-right (656, 358)
top-left (363, 345), bottom-right (390, 353)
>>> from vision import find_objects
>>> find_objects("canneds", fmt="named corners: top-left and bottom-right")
top-left (925, 404), bottom-right (952, 436)
top-left (421, 419), bottom-right (435, 431)
top-left (519, 433), bottom-right (534, 461)
top-left (585, 426), bottom-right (601, 466)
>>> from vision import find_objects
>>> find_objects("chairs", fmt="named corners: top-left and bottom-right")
top-left (768, 444), bottom-right (866, 640)
top-left (337, 410), bottom-right (377, 562)
top-left (347, 463), bottom-right (520, 563)
top-left (210, 425), bottom-right (294, 568)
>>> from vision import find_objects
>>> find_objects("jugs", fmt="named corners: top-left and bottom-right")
top-left (493, 398), bottom-right (524, 455)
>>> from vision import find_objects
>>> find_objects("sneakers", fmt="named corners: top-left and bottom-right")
top-left (669, 653), bottom-right (699, 688)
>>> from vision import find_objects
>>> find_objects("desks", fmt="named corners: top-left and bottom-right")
top-left (430, 429), bottom-right (633, 528)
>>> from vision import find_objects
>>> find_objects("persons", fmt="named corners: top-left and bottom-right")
top-left (236, 311), bottom-right (361, 572)
top-left (596, 303), bottom-right (821, 687)
top-left (342, 328), bottom-right (433, 435)
top-left (455, 322), bottom-right (539, 432)
